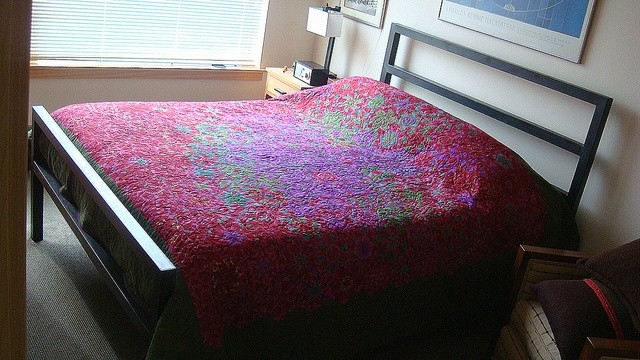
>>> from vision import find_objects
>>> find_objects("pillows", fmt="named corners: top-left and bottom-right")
top-left (583, 238), bottom-right (640, 325)
top-left (531, 275), bottom-right (627, 359)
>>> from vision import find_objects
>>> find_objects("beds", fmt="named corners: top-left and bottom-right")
top-left (31, 22), bottom-right (613, 346)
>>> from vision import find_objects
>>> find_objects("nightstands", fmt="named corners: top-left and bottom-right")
top-left (264, 65), bottom-right (340, 100)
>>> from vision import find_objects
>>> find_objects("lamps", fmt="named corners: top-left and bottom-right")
top-left (306, 3), bottom-right (342, 78)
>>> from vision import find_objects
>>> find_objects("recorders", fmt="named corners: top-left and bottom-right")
top-left (292, 59), bottom-right (329, 86)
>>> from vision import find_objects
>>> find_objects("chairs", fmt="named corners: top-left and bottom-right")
top-left (497, 239), bottom-right (638, 358)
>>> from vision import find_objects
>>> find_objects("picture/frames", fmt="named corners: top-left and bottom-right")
top-left (437, 0), bottom-right (595, 65)
top-left (339, 1), bottom-right (389, 29)
